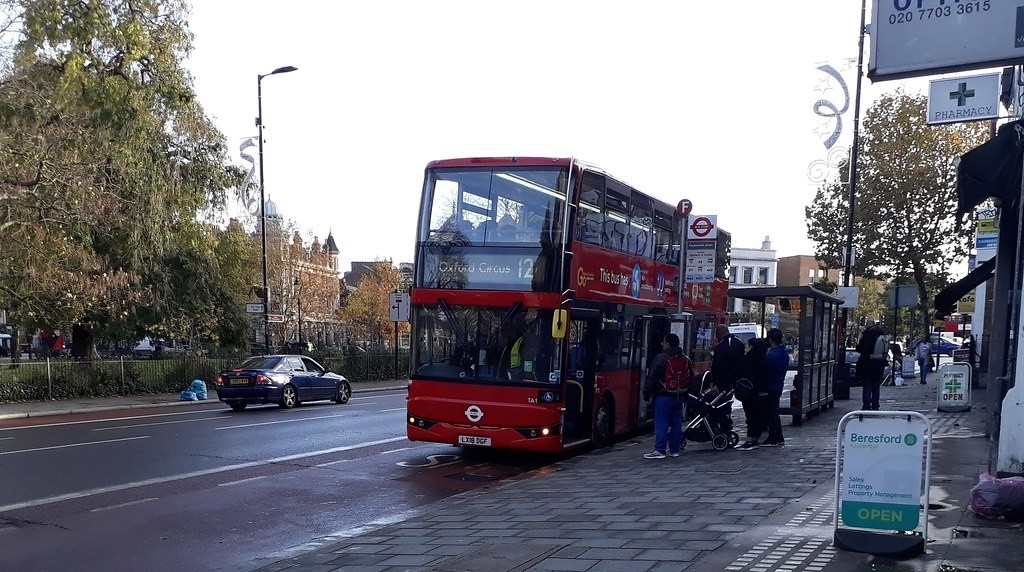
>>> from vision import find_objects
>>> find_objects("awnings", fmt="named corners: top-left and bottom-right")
top-left (954, 125), bottom-right (1017, 234)
top-left (934, 255), bottom-right (997, 319)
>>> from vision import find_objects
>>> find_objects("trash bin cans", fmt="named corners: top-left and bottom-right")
top-left (832, 362), bottom-right (851, 400)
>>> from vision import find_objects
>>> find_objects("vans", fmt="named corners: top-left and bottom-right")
top-left (731, 322), bottom-right (769, 353)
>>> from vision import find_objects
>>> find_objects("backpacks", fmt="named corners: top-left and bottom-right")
top-left (658, 353), bottom-right (691, 392)
top-left (869, 334), bottom-right (890, 360)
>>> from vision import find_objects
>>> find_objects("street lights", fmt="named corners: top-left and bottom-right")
top-left (258, 65), bottom-right (298, 352)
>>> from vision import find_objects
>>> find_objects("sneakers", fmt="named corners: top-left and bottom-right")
top-left (752, 440), bottom-right (759, 449)
top-left (736, 441), bottom-right (753, 450)
top-left (758, 437), bottom-right (778, 446)
top-left (665, 449), bottom-right (679, 457)
top-left (777, 435), bottom-right (785, 444)
top-left (644, 449), bottom-right (666, 458)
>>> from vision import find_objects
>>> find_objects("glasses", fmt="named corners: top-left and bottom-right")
top-left (661, 343), bottom-right (666, 345)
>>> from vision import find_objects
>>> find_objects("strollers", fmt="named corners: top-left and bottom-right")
top-left (678, 370), bottom-right (741, 453)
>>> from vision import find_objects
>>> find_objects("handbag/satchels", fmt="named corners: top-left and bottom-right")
top-left (970, 472), bottom-right (1024, 519)
top-left (925, 352), bottom-right (934, 367)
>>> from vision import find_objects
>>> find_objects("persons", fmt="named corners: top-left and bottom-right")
top-left (570, 331), bottom-right (588, 374)
top-left (855, 321), bottom-right (889, 411)
top-left (711, 323), bottom-right (790, 451)
top-left (501, 327), bottom-right (525, 381)
top-left (642, 334), bottom-right (695, 459)
top-left (915, 334), bottom-right (932, 384)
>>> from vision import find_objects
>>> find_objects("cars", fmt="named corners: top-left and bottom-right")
top-left (846, 346), bottom-right (902, 387)
top-left (216, 354), bottom-right (352, 411)
top-left (908, 331), bottom-right (971, 357)
top-left (782, 337), bottom-right (799, 368)
top-left (131, 334), bottom-right (187, 359)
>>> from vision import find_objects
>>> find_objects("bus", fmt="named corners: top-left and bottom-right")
top-left (407, 156), bottom-right (731, 465)
